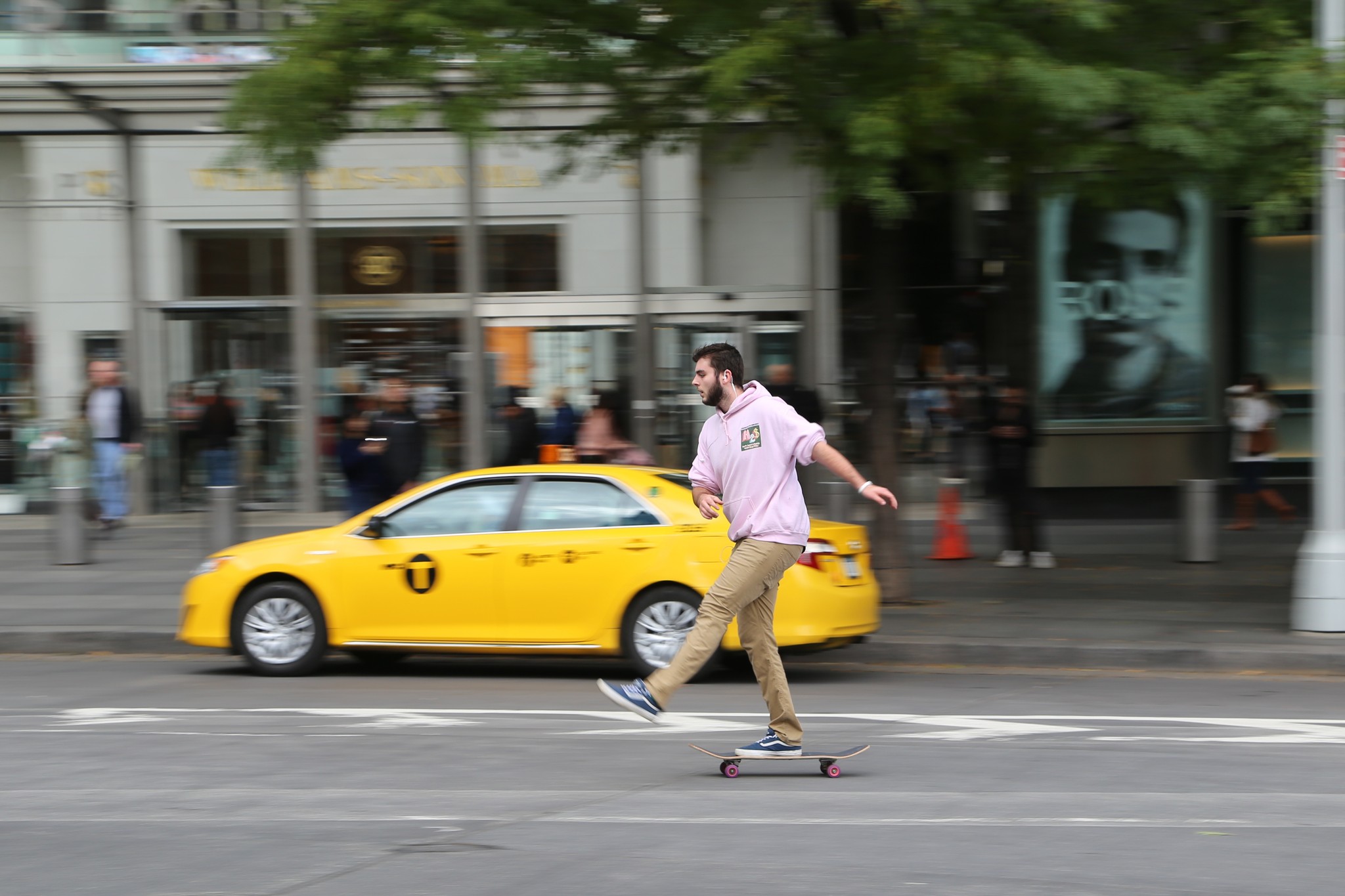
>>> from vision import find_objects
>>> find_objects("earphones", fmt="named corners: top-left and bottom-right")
top-left (723, 372), bottom-right (727, 380)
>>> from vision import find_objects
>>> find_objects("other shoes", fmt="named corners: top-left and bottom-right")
top-left (1030, 550), bottom-right (1059, 568)
top-left (100, 518), bottom-right (118, 528)
top-left (994, 549), bottom-right (1027, 567)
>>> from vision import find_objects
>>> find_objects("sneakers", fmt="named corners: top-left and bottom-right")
top-left (735, 729), bottom-right (803, 758)
top-left (596, 678), bottom-right (662, 722)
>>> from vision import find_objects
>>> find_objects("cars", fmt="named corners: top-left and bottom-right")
top-left (176, 463), bottom-right (880, 685)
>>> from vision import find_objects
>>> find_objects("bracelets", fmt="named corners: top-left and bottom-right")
top-left (857, 480), bottom-right (873, 493)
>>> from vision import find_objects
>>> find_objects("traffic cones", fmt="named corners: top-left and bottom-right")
top-left (925, 485), bottom-right (980, 561)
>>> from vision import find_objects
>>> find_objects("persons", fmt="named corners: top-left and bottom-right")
top-left (596, 340), bottom-right (901, 758)
top-left (1043, 185), bottom-right (1207, 418)
top-left (72, 357), bottom-right (1303, 567)
